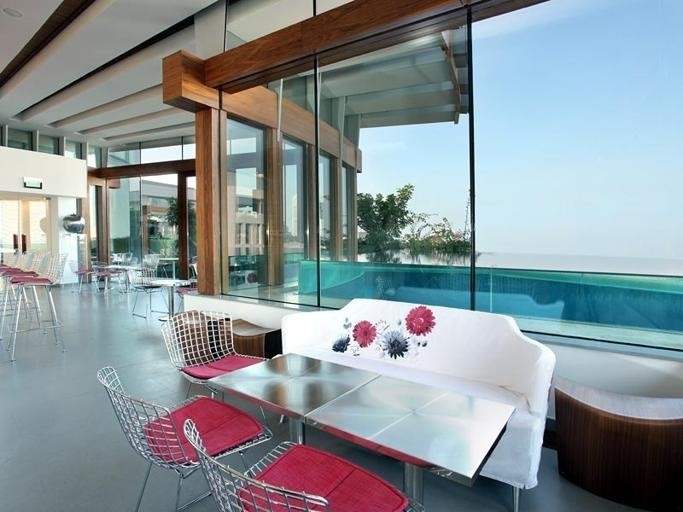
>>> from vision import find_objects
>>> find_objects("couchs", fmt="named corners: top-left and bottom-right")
top-left (279, 298), bottom-right (556, 511)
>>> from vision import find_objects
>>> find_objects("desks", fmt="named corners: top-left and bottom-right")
top-left (152, 280), bottom-right (191, 321)
top-left (305, 376), bottom-right (517, 507)
top-left (208, 351), bottom-right (380, 447)
top-left (95, 265), bottom-right (135, 289)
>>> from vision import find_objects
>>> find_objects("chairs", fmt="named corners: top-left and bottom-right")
top-left (183, 419), bottom-right (410, 512)
top-left (0, 264), bottom-right (11, 305)
top-left (97, 272), bottom-right (124, 296)
top-left (98, 367), bottom-right (272, 512)
top-left (177, 288), bottom-right (199, 313)
top-left (1, 250), bottom-right (18, 310)
top-left (2, 253), bottom-right (30, 318)
top-left (1, 251), bottom-right (59, 350)
top-left (69, 261), bottom-right (99, 293)
top-left (8, 253), bottom-right (70, 360)
top-left (160, 311), bottom-right (268, 424)
top-left (127, 269), bottom-right (169, 318)
